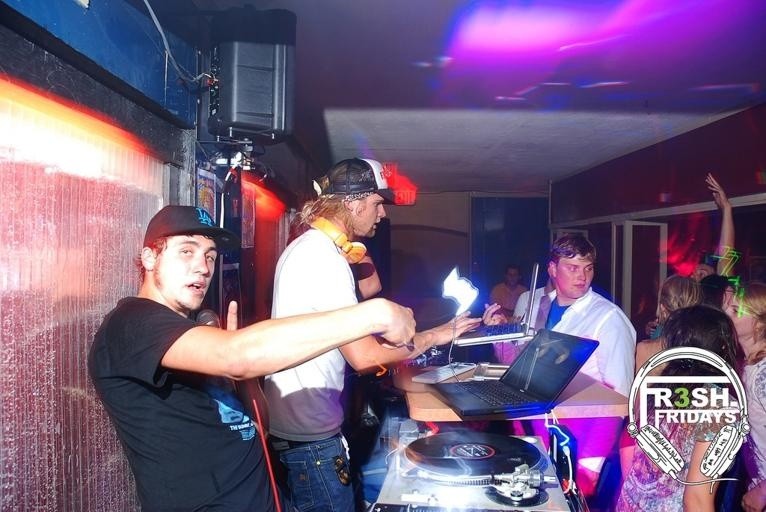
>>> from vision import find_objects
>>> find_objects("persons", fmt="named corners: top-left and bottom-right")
top-left (481, 171), bottom-right (766, 512)
top-left (87, 206), bottom-right (416, 512)
top-left (287, 200), bottom-right (382, 472)
top-left (260, 158), bottom-right (482, 512)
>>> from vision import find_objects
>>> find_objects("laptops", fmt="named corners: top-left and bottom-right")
top-left (452, 263), bottom-right (540, 346)
top-left (426, 327), bottom-right (600, 417)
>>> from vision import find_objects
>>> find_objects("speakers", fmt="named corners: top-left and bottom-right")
top-left (206, 8), bottom-right (297, 156)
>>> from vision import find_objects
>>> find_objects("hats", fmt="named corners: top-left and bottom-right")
top-left (323, 157), bottom-right (416, 206)
top-left (143, 205), bottom-right (240, 252)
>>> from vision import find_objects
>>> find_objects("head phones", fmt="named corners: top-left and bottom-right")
top-left (309, 216), bottom-right (367, 266)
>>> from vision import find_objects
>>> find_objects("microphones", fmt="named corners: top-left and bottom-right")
top-left (199, 310), bottom-right (221, 331)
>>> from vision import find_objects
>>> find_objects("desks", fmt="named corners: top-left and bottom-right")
top-left (390, 363), bottom-right (629, 436)
